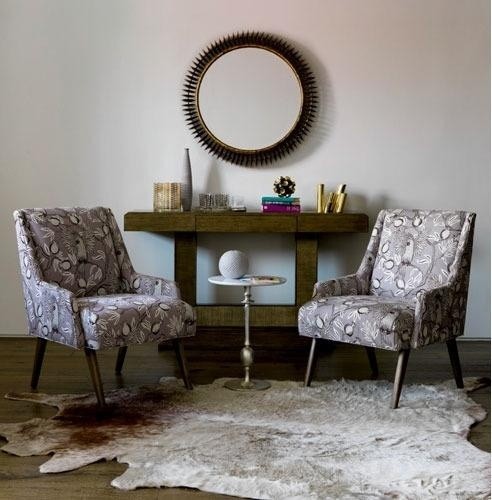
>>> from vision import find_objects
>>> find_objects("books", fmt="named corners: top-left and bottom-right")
top-left (263, 205), bottom-right (300, 213)
top-left (262, 196), bottom-right (300, 206)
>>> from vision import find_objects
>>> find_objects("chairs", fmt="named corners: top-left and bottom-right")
top-left (12, 206), bottom-right (198, 417)
top-left (296, 208), bottom-right (476, 409)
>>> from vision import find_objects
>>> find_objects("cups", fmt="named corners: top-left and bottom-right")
top-left (232, 195), bottom-right (247, 212)
top-left (213, 193), bottom-right (230, 211)
top-left (153, 181), bottom-right (184, 214)
top-left (198, 193), bottom-right (214, 211)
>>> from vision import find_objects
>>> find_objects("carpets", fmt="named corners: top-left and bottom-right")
top-left (1, 374), bottom-right (490, 500)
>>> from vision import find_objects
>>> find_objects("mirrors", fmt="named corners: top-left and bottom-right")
top-left (182, 29), bottom-right (319, 168)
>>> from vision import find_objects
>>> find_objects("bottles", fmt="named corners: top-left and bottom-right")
top-left (180, 148), bottom-right (193, 211)
top-left (317, 182), bottom-right (348, 214)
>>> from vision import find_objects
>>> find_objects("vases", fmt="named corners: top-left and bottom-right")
top-left (174, 148), bottom-right (192, 211)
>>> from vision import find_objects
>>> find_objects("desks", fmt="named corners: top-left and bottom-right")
top-left (123, 210), bottom-right (368, 362)
